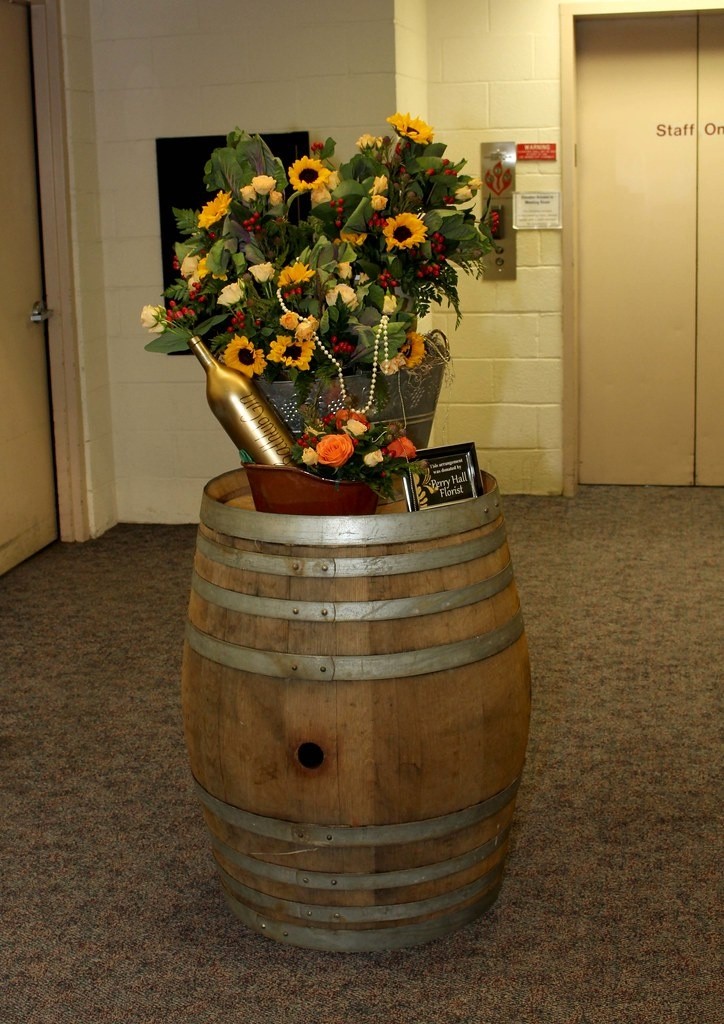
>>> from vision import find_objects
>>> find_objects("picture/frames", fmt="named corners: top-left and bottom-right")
top-left (401, 440), bottom-right (483, 512)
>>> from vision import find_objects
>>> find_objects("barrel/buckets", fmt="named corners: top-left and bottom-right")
top-left (184, 456), bottom-right (531, 954)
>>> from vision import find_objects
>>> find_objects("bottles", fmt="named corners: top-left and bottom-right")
top-left (186, 334), bottom-right (305, 469)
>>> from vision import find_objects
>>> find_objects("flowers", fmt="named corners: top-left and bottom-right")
top-left (293, 411), bottom-right (415, 494)
top-left (136, 112), bottom-right (503, 379)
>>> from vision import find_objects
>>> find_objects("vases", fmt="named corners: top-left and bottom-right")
top-left (244, 354), bottom-right (449, 459)
top-left (241, 462), bottom-right (378, 515)
